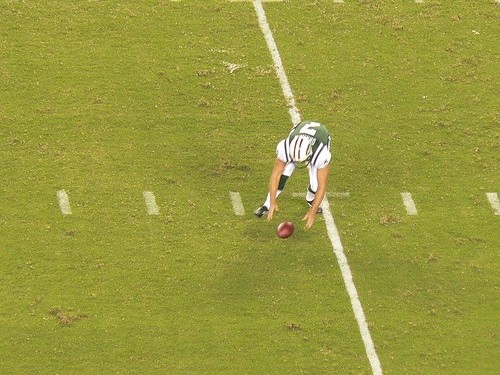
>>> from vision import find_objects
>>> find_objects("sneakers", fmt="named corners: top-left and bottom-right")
top-left (254, 206), bottom-right (269, 218)
top-left (307, 201), bottom-right (322, 213)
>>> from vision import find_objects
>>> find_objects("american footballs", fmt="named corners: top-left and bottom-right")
top-left (276, 221), bottom-right (294, 240)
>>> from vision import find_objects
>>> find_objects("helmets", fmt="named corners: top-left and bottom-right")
top-left (289, 136), bottom-right (312, 162)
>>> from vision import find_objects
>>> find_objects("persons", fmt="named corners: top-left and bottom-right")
top-left (254, 120), bottom-right (332, 231)
